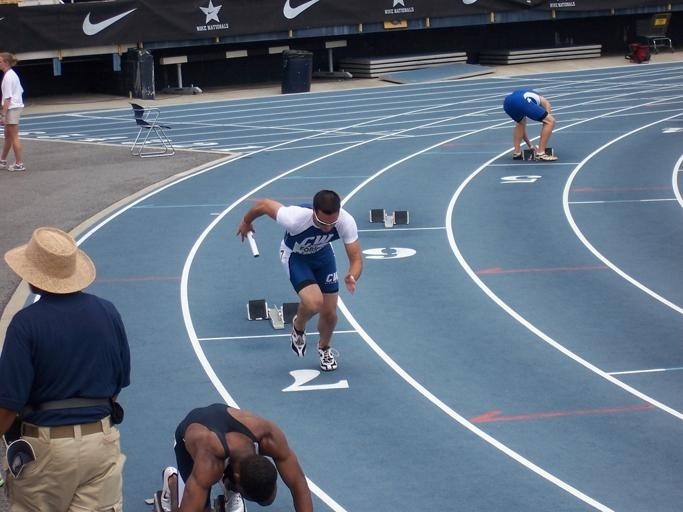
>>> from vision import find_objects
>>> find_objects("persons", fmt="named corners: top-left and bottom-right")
top-left (236, 189), bottom-right (363, 372)
top-left (0, 227), bottom-right (131, 512)
top-left (503, 88), bottom-right (558, 161)
top-left (0, 52), bottom-right (26, 171)
top-left (160, 402), bottom-right (313, 512)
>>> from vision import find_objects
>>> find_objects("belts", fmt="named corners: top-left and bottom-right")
top-left (23, 421), bottom-right (102, 438)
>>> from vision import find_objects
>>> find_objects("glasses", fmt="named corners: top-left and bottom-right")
top-left (314, 209), bottom-right (339, 226)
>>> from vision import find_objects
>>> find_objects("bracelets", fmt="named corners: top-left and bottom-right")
top-left (1, 114), bottom-right (6, 117)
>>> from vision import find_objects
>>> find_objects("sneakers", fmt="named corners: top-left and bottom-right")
top-left (0, 160), bottom-right (8, 168)
top-left (8, 163), bottom-right (26, 172)
top-left (161, 466), bottom-right (178, 512)
top-left (535, 152), bottom-right (558, 161)
top-left (218, 472), bottom-right (247, 512)
top-left (291, 314), bottom-right (306, 357)
top-left (317, 340), bottom-right (340, 371)
top-left (513, 152), bottom-right (523, 160)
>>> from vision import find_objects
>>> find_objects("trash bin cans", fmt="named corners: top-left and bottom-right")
top-left (281, 50), bottom-right (313, 93)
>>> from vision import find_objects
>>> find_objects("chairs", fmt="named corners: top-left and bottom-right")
top-left (128, 105), bottom-right (175, 159)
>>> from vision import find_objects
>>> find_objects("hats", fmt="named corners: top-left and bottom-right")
top-left (4, 227), bottom-right (96, 294)
top-left (240, 456), bottom-right (277, 506)
